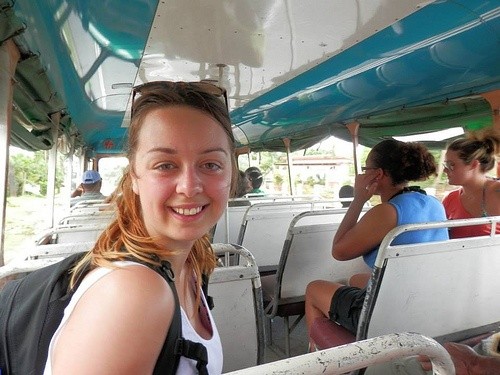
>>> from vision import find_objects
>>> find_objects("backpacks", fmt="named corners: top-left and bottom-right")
top-left (0, 251), bottom-right (208, 375)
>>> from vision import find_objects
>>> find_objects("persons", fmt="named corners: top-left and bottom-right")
top-left (206, 128), bottom-right (500, 375)
top-left (43, 81), bottom-right (238, 375)
top-left (70, 170), bottom-right (107, 208)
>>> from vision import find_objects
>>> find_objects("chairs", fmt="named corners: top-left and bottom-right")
top-left (0, 192), bottom-right (500, 375)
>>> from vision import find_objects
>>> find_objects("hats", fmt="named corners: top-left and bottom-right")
top-left (81, 169), bottom-right (101, 184)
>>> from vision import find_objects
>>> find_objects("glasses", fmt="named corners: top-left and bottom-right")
top-left (130, 79), bottom-right (229, 120)
top-left (442, 160), bottom-right (474, 171)
top-left (361, 167), bottom-right (388, 176)
